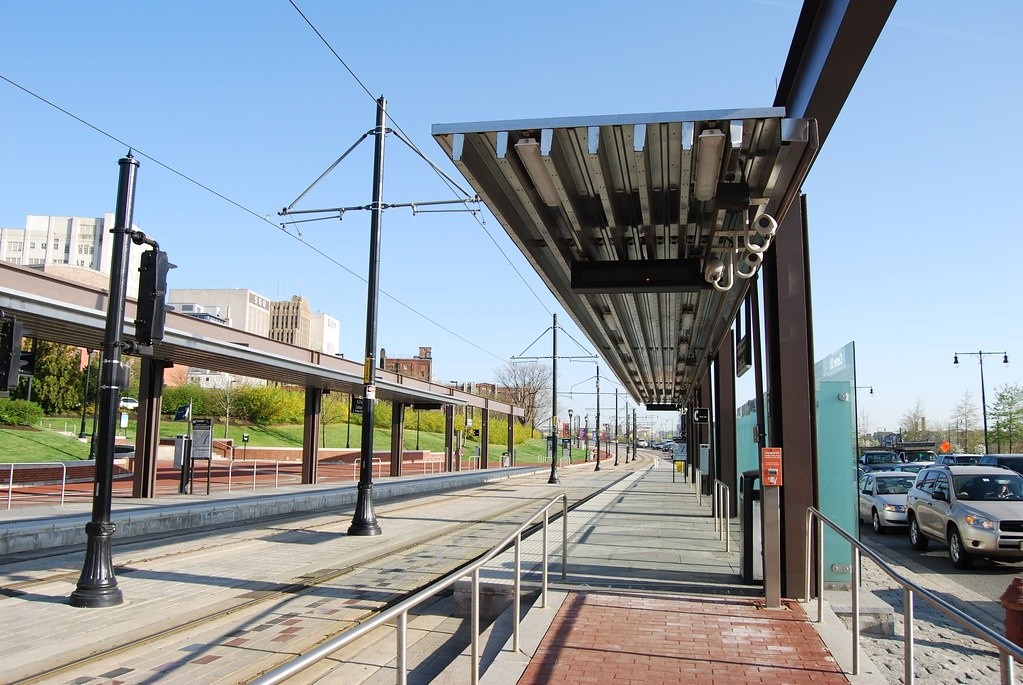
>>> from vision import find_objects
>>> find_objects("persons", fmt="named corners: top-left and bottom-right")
top-left (990, 484), bottom-right (1009, 497)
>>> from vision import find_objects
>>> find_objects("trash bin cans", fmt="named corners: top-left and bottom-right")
top-left (501, 452), bottom-right (511, 467)
top-left (739, 469), bottom-right (763, 586)
top-left (173, 434), bottom-right (194, 470)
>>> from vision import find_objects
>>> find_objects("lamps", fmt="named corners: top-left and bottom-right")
top-left (322, 387), bottom-right (331, 395)
top-left (674, 342), bottom-right (690, 402)
top-left (602, 311), bottom-right (618, 330)
top-left (692, 128), bottom-right (727, 202)
top-left (681, 310), bottom-right (694, 331)
top-left (513, 139), bottom-right (562, 206)
top-left (617, 340), bottom-right (650, 403)
top-left (162, 359), bottom-right (174, 368)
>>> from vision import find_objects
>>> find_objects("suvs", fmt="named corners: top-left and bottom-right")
top-left (978, 454), bottom-right (1023, 491)
top-left (636, 438), bottom-right (648, 448)
top-left (907, 463), bottom-right (1023, 569)
top-left (935, 455), bottom-right (984, 466)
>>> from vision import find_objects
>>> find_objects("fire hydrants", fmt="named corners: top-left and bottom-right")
top-left (997, 576), bottom-right (1023, 662)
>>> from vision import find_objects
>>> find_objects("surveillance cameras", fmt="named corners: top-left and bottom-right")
top-left (705, 214), bottom-right (778, 283)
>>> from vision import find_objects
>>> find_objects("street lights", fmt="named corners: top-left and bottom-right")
top-left (450, 380), bottom-right (457, 389)
top-left (224, 380), bottom-right (236, 439)
top-left (956, 419), bottom-right (963, 447)
top-left (876, 427), bottom-right (886, 442)
top-left (584, 413), bottom-right (589, 463)
top-left (953, 351), bottom-right (1012, 453)
top-left (568, 409), bottom-right (585, 462)
top-left (336, 353), bottom-right (343, 359)
top-left (79, 347), bottom-right (93, 438)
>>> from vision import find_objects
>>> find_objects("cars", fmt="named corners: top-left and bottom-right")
top-left (119, 398), bottom-right (138, 411)
top-left (898, 451), bottom-right (940, 463)
top-left (854, 471), bottom-right (918, 532)
top-left (858, 453), bottom-right (903, 480)
top-left (891, 462), bottom-right (935, 487)
top-left (648, 439), bottom-right (673, 452)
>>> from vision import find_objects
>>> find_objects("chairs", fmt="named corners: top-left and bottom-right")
top-left (877, 479), bottom-right (890, 494)
top-left (903, 482), bottom-right (914, 490)
